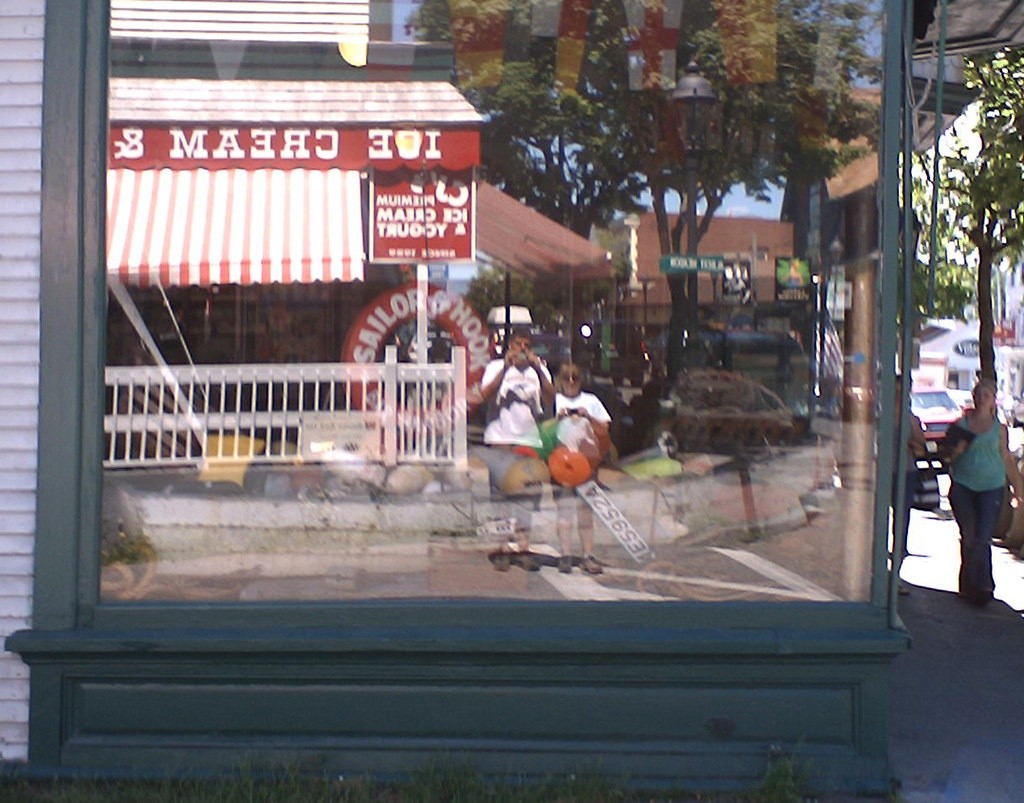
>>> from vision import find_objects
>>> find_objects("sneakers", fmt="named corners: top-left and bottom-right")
top-left (897, 581), bottom-right (911, 594)
top-left (559, 555), bottom-right (573, 573)
top-left (579, 556), bottom-right (603, 574)
top-left (493, 552), bottom-right (510, 572)
top-left (509, 551), bottom-right (541, 571)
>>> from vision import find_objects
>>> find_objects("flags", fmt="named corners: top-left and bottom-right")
top-left (365, 0), bottom-right (843, 169)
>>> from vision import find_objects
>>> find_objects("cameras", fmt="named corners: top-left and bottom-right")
top-left (515, 353), bottom-right (530, 362)
top-left (567, 408), bottom-right (578, 415)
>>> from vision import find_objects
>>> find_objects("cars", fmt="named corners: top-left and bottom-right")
top-left (911, 386), bottom-right (964, 470)
top-left (628, 366), bottom-right (807, 453)
top-left (919, 319), bottom-right (1011, 389)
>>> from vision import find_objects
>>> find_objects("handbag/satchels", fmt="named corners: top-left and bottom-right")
top-left (910, 442), bottom-right (941, 510)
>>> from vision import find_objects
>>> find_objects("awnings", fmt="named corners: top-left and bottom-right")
top-left (475, 175), bottom-right (618, 281)
top-left (106, 167), bottom-right (368, 287)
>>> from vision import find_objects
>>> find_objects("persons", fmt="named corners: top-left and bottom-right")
top-left (481, 328), bottom-right (556, 571)
top-left (936, 379), bottom-right (1022, 606)
top-left (548, 361), bottom-right (612, 573)
top-left (886, 374), bottom-right (924, 594)
